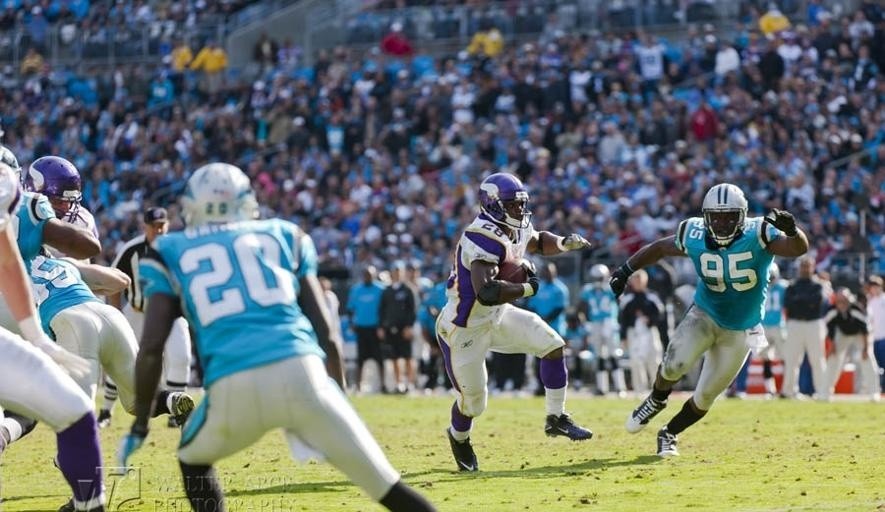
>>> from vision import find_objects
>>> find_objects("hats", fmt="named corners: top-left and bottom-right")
top-left (143, 206), bottom-right (169, 224)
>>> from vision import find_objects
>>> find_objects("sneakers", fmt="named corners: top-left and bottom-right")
top-left (657, 424), bottom-right (680, 459)
top-left (544, 414), bottom-right (592, 442)
top-left (58, 495), bottom-right (105, 511)
top-left (447, 427), bottom-right (478, 471)
top-left (166, 392), bottom-right (194, 430)
top-left (96, 407), bottom-right (112, 430)
top-left (625, 393), bottom-right (668, 433)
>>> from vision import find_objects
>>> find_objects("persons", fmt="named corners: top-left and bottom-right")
top-left (0, 402), bottom-right (198, 511)
top-left (608, 182), bottom-right (809, 460)
top-left (435, 170), bottom-right (593, 471)
top-left (116, 161), bottom-right (441, 510)
top-left (0, 1), bottom-right (885, 402)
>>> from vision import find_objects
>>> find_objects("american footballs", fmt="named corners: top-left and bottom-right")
top-left (498, 257), bottom-right (532, 283)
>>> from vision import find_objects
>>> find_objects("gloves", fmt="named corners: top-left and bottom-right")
top-left (520, 261), bottom-right (540, 298)
top-left (764, 208), bottom-right (798, 239)
top-left (609, 260), bottom-right (634, 299)
top-left (18, 315), bottom-right (91, 379)
top-left (118, 424), bottom-right (150, 474)
top-left (556, 233), bottom-right (592, 252)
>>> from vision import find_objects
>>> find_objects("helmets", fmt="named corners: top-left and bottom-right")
top-left (178, 162), bottom-right (259, 226)
top-left (478, 172), bottom-right (533, 229)
top-left (0, 144), bottom-right (23, 186)
top-left (701, 182), bottom-right (749, 246)
top-left (22, 155), bottom-right (82, 224)
